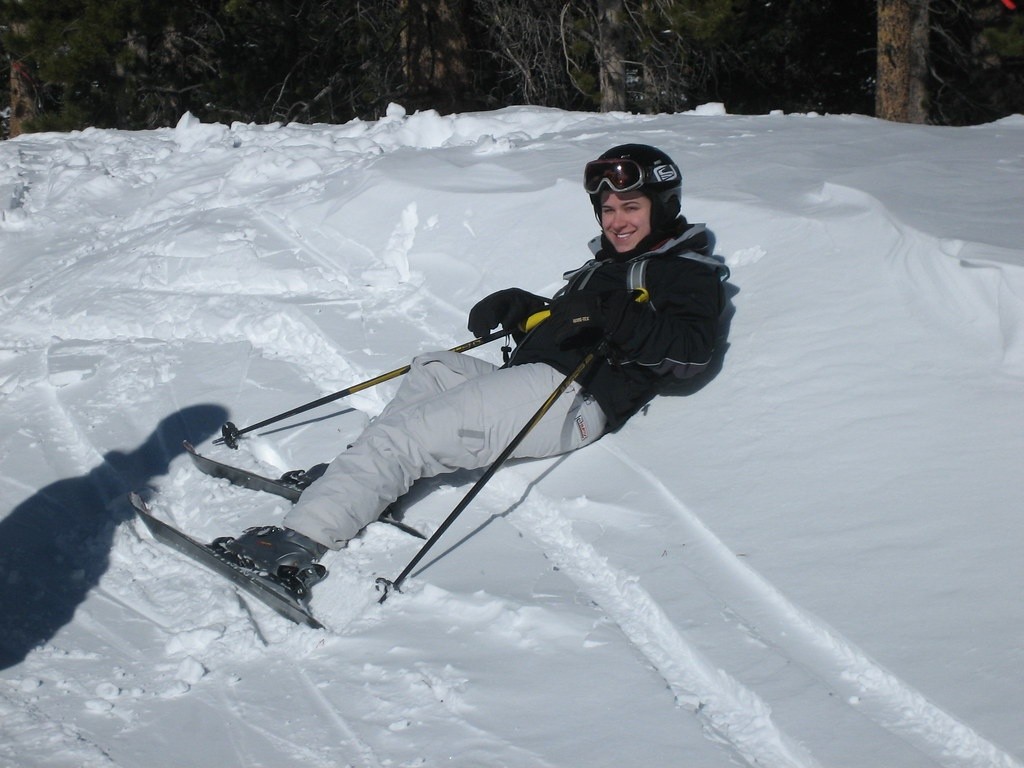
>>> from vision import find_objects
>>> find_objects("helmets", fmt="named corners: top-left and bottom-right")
top-left (588, 144), bottom-right (683, 230)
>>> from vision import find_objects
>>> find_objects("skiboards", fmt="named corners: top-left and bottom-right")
top-left (117, 424), bottom-right (441, 640)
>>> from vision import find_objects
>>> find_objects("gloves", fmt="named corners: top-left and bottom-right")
top-left (468, 287), bottom-right (534, 338)
top-left (550, 287), bottom-right (628, 351)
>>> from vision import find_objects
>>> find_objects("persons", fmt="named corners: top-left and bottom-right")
top-left (217, 145), bottom-right (729, 599)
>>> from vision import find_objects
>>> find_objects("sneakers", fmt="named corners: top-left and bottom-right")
top-left (226, 526), bottom-right (318, 582)
top-left (300, 463), bottom-right (330, 484)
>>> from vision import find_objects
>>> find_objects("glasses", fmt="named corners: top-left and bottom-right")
top-left (584, 159), bottom-right (681, 194)
